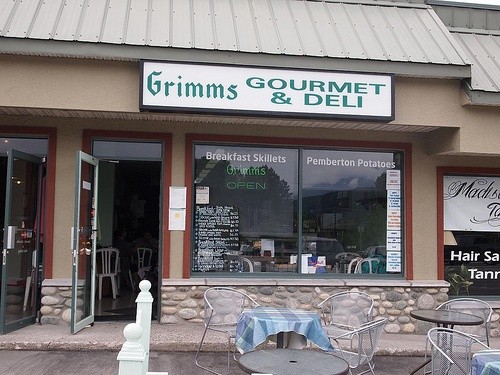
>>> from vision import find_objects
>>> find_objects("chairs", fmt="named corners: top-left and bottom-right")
top-left (195, 287), bottom-right (260, 375)
top-left (310, 291), bottom-right (375, 375)
top-left (96, 247), bottom-right (152, 301)
top-left (424, 327), bottom-right (491, 375)
top-left (324, 318), bottom-right (388, 375)
top-left (238, 252), bottom-right (386, 274)
top-left (435, 298), bottom-right (492, 347)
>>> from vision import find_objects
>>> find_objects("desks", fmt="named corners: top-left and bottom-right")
top-left (238, 348), bottom-right (349, 375)
top-left (471, 349), bottom-right (500, 375)
top-left (235, 308), bottom-right (335, 355)
top-left (410, 309), bottom-right (484, 375)
